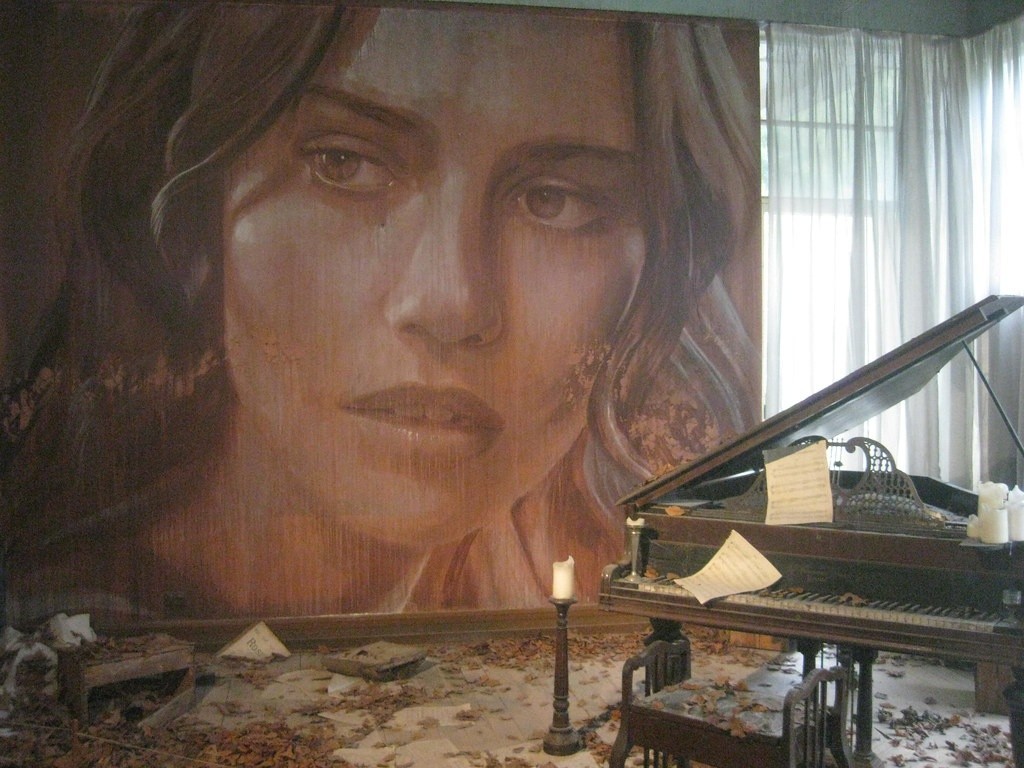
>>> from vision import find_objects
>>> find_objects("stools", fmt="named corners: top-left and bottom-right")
top-left (608, 639), bottom-right (862, 768)
top-left (67, 633), bottom-right (196, 720)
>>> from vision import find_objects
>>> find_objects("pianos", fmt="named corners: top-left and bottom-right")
top-left (598, 293), bottom-right (1024, 768)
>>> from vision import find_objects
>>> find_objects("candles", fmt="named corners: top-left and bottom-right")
top-left (552, 556), bottom-right (576, 599)
top-left (967, 481), bottom-right (1024, 544)
